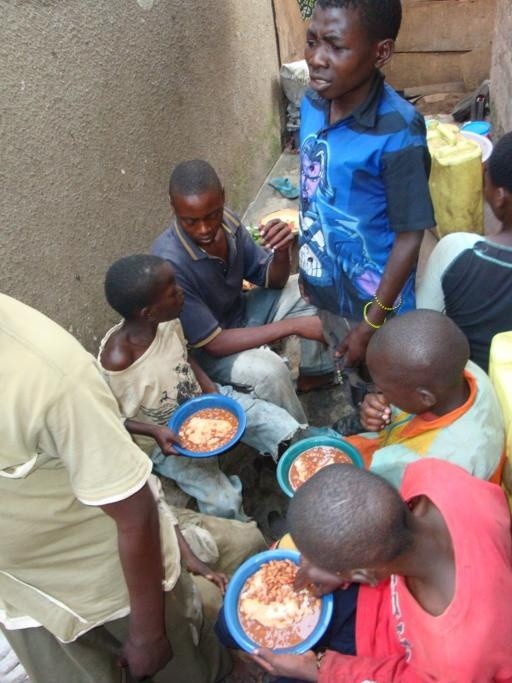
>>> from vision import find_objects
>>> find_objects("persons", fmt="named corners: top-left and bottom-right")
top-left (295, 0), bottom-right (434, 437)
top-left (149, 475), bottom-right (271, 683)
top-left (148, 159), bottom-right (338, 427)
top-left (354, 309), bottom-right (508, 494)
top-left (415, 132), bottom-right (512, 374)
top-left (0, 293), bottom-right (229, 683)
top-left (248, 457), bottom-right (512, 683)
top-left (95, 254), bottom-right (309, 523)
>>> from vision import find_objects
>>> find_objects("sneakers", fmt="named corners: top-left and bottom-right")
top-left (333, 412), bottom-right (367, 436)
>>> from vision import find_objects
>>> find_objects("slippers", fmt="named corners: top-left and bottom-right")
top-left (253, 496), bottom-right (287, 539)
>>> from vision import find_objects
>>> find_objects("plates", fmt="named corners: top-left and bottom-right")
top-left (275, 434), bottom-right (363, 500)
top-left (169, 394), bottom-right (248, 458)
top-left (222, 549), bottom-right (336, 656)
top-left (262, 208), bottom-right (300, 237)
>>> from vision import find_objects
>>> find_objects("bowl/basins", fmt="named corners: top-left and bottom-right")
top-left (463, 118), bottom-right (495, 137)
